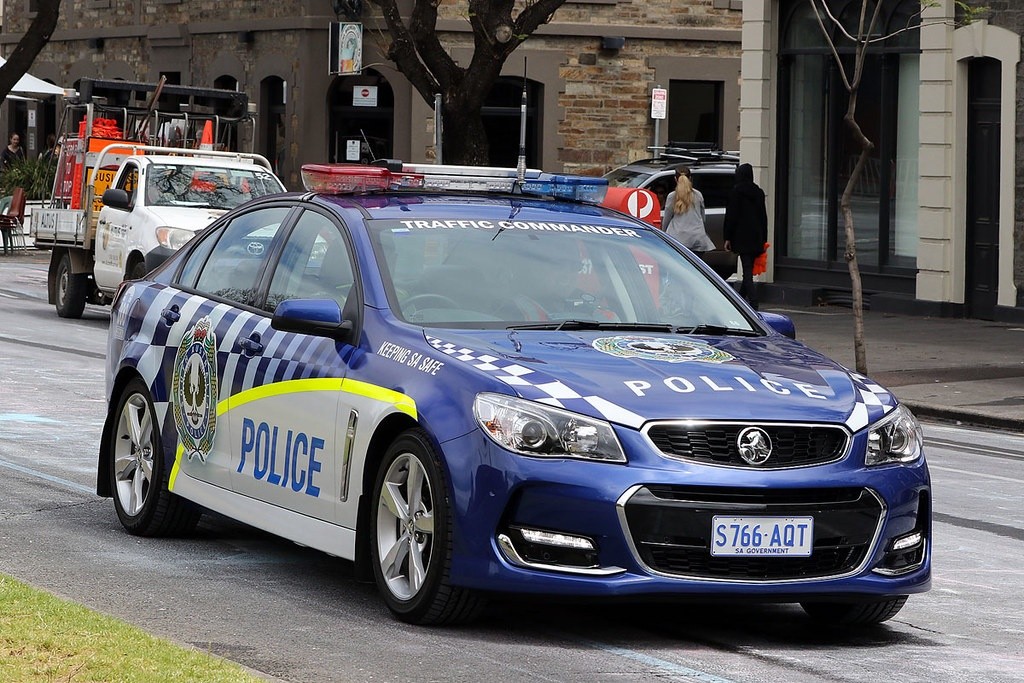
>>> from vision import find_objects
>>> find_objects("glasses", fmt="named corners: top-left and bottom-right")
top-left (654, 191), bottom-right (664, 195)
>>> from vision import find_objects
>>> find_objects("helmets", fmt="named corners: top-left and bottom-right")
top-left (520, 235), bottom-right (592, 302)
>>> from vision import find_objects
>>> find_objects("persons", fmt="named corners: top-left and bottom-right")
top-left (723, 163), bottom-right (770, 307)
top-left (38, 134), bottom-right (57, 160)
top-left (661, 166), bottom-right (717, 317)
top-left (520, 250), bottom-right (600, 321)
top-left (323, 234), bottom-right (416, 324)
top-left (0, 132), bottom-right (24, 171)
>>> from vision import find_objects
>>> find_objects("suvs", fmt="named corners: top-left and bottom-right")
top-left (597, 140), bottom-right (740, 271)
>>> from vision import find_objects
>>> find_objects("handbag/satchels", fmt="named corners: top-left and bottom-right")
top-left (750, 243), bottom-right (770, 276)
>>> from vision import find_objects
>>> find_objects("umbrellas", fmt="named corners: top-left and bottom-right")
top-left (0, 56), bottom-right (75, 103)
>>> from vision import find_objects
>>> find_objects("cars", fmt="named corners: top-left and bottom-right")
top-left (94, 151), bottom-right (931, 633)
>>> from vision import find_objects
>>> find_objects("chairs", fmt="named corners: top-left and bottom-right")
top-left (316, 245), bottom-right (352, 296)
top-left (415, 262), bottom-right (467, 307)
top-left (0, 188), bottom-right (27, 255)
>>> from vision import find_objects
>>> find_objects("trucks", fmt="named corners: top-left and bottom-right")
top-left (28, 73), bottom-right (290, 317)
